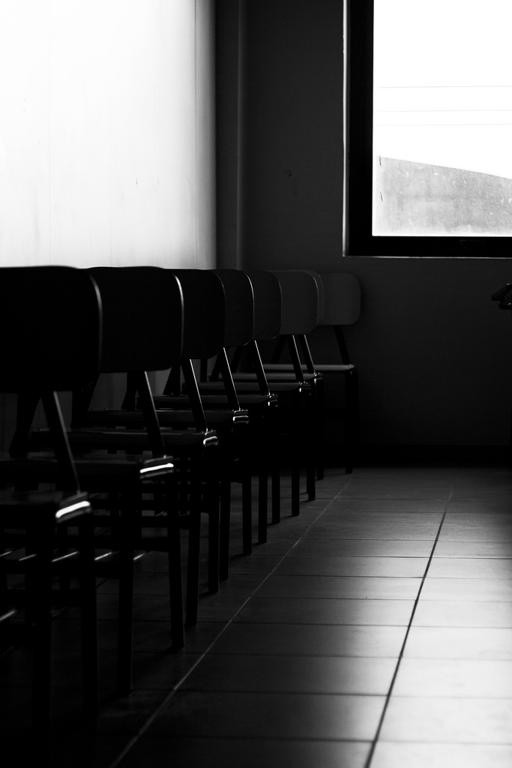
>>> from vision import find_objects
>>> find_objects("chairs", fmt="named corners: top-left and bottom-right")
top-left (0, 265), bottom-right (367, 768)
